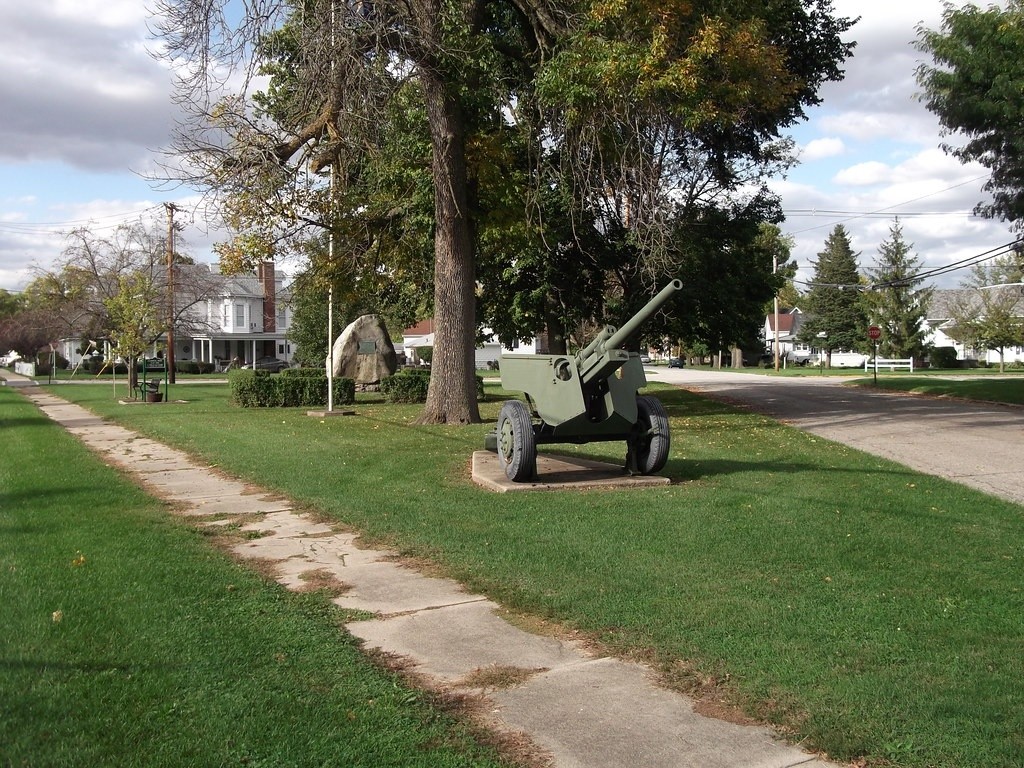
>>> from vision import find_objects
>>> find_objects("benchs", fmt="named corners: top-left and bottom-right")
top-left (133, 378), bottom-right (162, 402)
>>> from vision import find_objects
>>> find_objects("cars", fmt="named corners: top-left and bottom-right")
top-left (668, 359), bottom-right (683, 368)
top-left (639, 355), bottom-right (650, 363)
top-left (240, 356), bottom-right (288, 374)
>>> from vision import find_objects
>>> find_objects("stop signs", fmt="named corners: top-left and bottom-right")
top-left (868, 327), bottom-right (881, 339)
